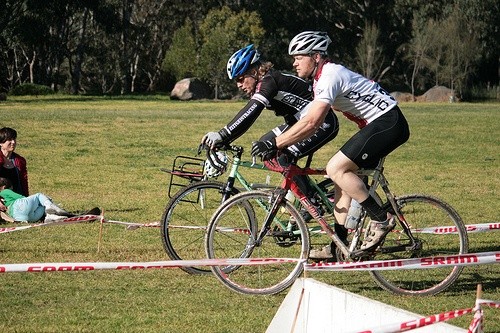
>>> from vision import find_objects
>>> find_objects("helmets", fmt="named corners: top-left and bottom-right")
top-left (205, 151), bottom-right (227, 178)
top-left (227, 43), bottom-right (260, 80)
top-left (288, 31), bottom-right (332, 55)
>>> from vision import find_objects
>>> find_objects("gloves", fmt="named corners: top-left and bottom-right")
top-left (250, 138), bottom-right (277, 158)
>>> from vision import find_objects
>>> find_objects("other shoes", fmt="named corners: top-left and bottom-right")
top-left (44, 204), bottom-right (66, 214)
top-left (43, 213), bottom-right (67, 223)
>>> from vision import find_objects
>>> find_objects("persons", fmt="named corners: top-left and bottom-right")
top-left (201, 44), bottom-right (339, 225)
top-left (251, 31), bottom-right (410, 259)
top-left (0, 127), bottom-right (29, 225)
top-left (0, 177), bottom-right (100, 223)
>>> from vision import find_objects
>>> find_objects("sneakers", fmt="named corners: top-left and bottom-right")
top-left (308, 244), bottom-right (339, 260)
top-left (289, 199), bottom-right (323, 224)
top-left (361, 213), bottom-right (396, 251)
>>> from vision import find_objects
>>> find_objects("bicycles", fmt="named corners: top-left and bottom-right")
top-left (158, 134), bottom-right (387, 276)
top-left (202, 140), bottom-right (470, 298)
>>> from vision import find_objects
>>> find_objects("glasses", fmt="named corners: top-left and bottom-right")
top-left (236, 69), bottom-right (252, 84)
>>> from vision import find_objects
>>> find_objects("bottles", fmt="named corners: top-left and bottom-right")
top-left (281, 188), bottom-right (296, 214)
top-left (344, 175), bottom-right (370, 230)
